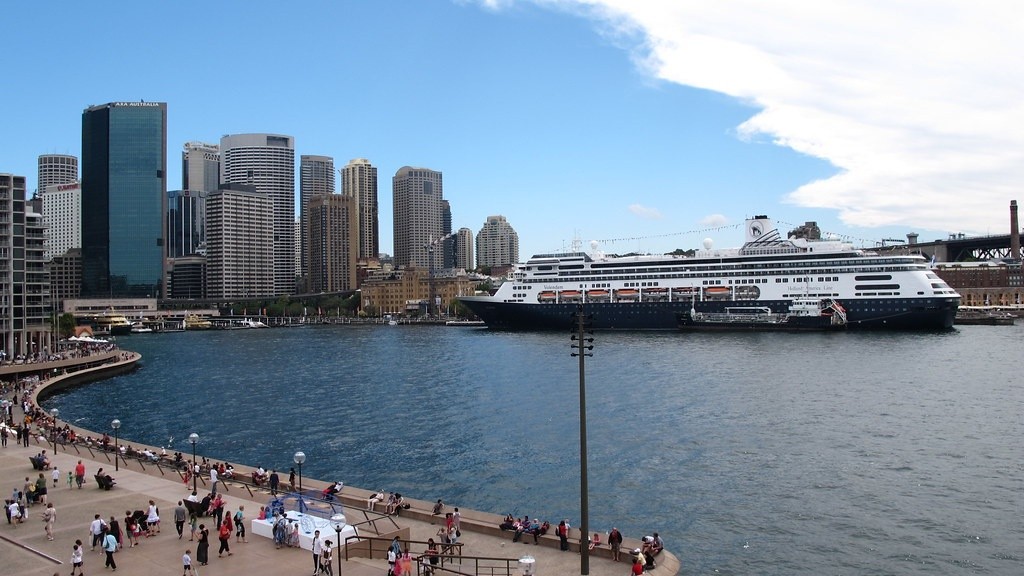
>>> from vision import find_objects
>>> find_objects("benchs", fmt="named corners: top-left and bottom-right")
top-left (94, 475), bottom-right (116, 490)
top-left (29, 456), bottom-right (47, 470)
top-left (183, 498), bottom-right (205, 516)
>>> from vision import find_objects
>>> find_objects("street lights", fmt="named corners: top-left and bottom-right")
top-left (111, 419), bottom-right (121, 471)
top-left (330, 513), bottom-right (347, 576)
top-left (293, 451), bottom-right (305, 512)
top-left (189, 433), bottom-right (199, 494)
top-left (51, 408), bottom-right (59, 454)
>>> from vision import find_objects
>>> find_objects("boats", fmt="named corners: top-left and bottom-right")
top-left (97, 304), bottom-right (132, 335)
top-left (954, 309), bottom-right (1016, 326)
top-left (674, 283), bottom-right (847, 333)
top-left (186, 313), bottom-right (212, 329)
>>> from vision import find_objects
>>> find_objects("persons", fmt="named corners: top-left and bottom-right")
top-left (589, 533), bottom-right (601, 550)
top-left (368, 489), bottom-right (384, 511)
top-left (269, 469), bottom-right (279, 495)
top-left (431, 499), bottom-right (444, 517)
top-left (631, 533), bottom-right (664, 576)
top-left (384, 492), bottom-right (405, 517)
top-left (312, 530), bottom-right (324, 576)
top-left (387, 508), bottom-right (460, 576)
top-left (504, 514), bottom-right (571, 552)
top-left (323, 540), bottom-right (333, 576)
top-left (0, 341), bottom-right (135, 361)
top-left (321, 482), bottom-right (343, 502)
top-left (608, 527), bottom-right (622, 561)
top-left (578, 528), bottom-right (591, 555)
top-left (289, 467), bottom-right (296, 492)
top-left (251, 466), bottom-right (269, 485)
top-left (0, 367), bottom-right (234, 576)
top-left (235, 505), bottom-right (248, 543)
top-left (257, 502), bottom-right (300, 549)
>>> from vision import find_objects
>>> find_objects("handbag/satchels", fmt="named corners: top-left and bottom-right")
top-left (320, 557), bottom-right (329, 565)
top-left (130, 525), bottom-right (136, 530)
top-left (101, 525), bottom-right (107, 531)
top-left (405, 504), bottom-right (411, 509)
top-left (45, 515), bottom-right (51, 522)
top-left (370, 494), bottom-right (376, 499)
top-left (17, 511), bottom-right (21, 517)
top-left (456, 530), bottom-right (461, 537)
top-left (225, 531), bottom-right (230, 540)
top-left (396, 551), bottom-right (402, 558)
top-left (555, 527), bottom-right (560, 536)
top-left (238, 524), bottom-right (243, 532)
top-left (24, 508), bottom-right (29, 519)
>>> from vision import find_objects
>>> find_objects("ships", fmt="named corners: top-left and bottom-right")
top-left (457, 215), bottom-right (963, 333)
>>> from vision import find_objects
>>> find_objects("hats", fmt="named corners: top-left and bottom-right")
top-left (338, 482), bottom-right (343, 484)
